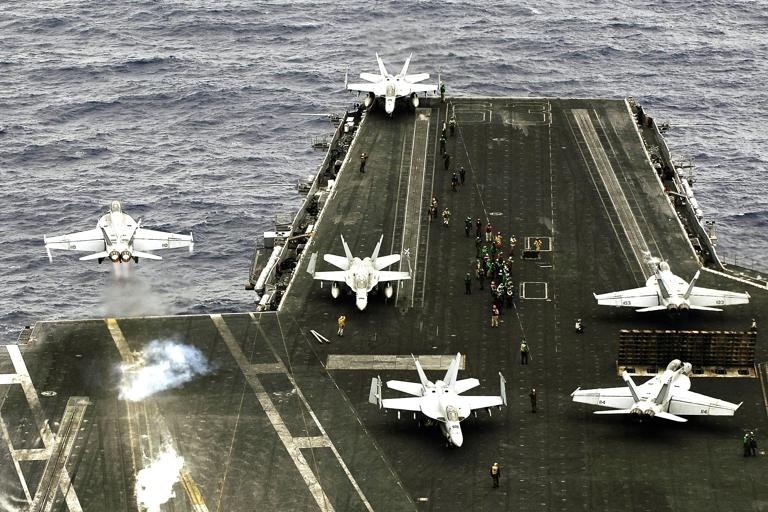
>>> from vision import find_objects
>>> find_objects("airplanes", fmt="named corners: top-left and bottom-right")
top-left (346, 51), bottom-right (441, 115)
top-left (594, 261), bottom-right (751, 313)
top-left (568, 358), bottom-right (744, 421)
top-left (375, 352), bottom-right (508, 450)
top-left (314, 232), bottom-right (413, 311)
top-left (40, 200), bottom-right (194, 268)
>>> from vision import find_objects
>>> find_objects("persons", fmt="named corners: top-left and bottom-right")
top-left (440, 83), bottom-right (447, 101)
top-left (519, 339), bottom-right (529, 365)
top-left (575, 319), bottom-right (586, 334)
top-left (742, 432), bottom-right (758, 457)
top-left (490, 462), bottom-right (501, 488)
top-left (427, 194), bottom-right (517, 329)
top-left (438, 115), bottom-right (466, 189)
top-left (336, 315), bottom-right (346, 336)
top-left (533, 237), bottom-right (543, 251)
top-left (359, 156), bottom-right (366, 173)
top-left (528, 386), bottom-right (536, 413)
top-left (750, 319), bottom-right (757, 330)
